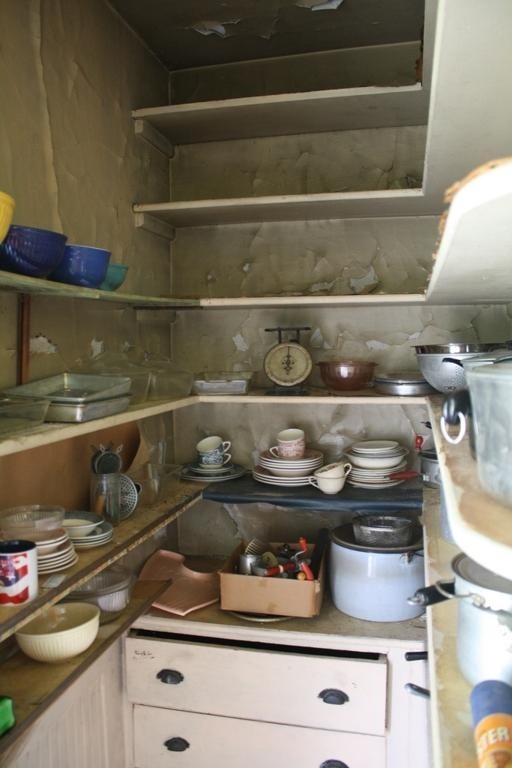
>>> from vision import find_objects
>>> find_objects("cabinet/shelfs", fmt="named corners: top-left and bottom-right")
top-left (0, 111), bottom-right (512, 768)
top-left (119, 590), bottom-right (430, 768)
top-left (0, 631), bottom-right (119, 766)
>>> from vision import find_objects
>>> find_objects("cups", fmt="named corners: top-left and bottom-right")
top-left (201, 455), bottom-right (231, 465)
top-left (308, 478), bottom-right (345, 495)
top-left (269, 446), bottom-right (306, 459)
top-left (276, 428), bottom-right (305, 446)
top-left (0, 540), bottom-right (38, 608)
top-left (196, 435), bottom-right (231, 454)
top-left (315, 462), bottom-right (352, 478)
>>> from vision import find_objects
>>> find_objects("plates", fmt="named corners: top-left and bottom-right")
top-left (230, 610), bottom-right (294, 623)
top-left (352, 441), bottom-right (403, 458)
top-left (341, 460), bottom-right (408, 490)
top-left (200, 464), bottom-right (223, 469)
top-left (3, 372), bottom-right (132, 402)
top-left (182, 467), bottom-right (244, 483)
top-left (36, 529), bottom-right (67, 554)
top-left (71, 522), bottom-right (114, 551)
top-left (193, 370), bottom-right (257, 395)
top-left (45, 393), bottom-right (133, 423)
top-left (137, 549), bottom-right (221, 617)
top-left (189, 465), bottom-right (233, 476)
top-left (37, 541), bottom-right (79, 575)
top-left (253, 466), bottom-right (316, 488)
top-left (257, 449), bottom-right (323, 477)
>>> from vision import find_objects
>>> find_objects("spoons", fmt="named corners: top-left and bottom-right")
top-left (94, 452), bottom-right (123, 516)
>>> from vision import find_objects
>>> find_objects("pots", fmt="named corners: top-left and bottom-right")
top-left (329, 524), bottom-right (427, 625)
top-left (436, 553), bottom-right (512, 686)
top-left (443, 346), bottom-right (512, 371)
top-left (418, 354), bottom-right (477, 394)
top-left (417, 447), bottom-right (442, 489)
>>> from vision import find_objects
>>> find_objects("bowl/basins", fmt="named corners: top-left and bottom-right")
top-left (2, 191), bottom-right (15, 245)
top-left (2, 223), bottom-right (68, 281)
top-left (64, 569), bottom-right (132, 626)
top-left (411, 344), bottom-right (504, 353)
top-left (352, 515), bottom-right (413, 547)
top-left (344, 447), bottom-right (410, 469)
top-left (101, 262), bottom-right (129, 289)
top-left (14, 602), bottom-right (101, 665)
top-left (1, 504), bottom-right (66, 540)
top-left (64, 510), bottom-right (105, 537)
top-left (314, 361), bottom-right (379, 390)
top-left (59, 243), bottom-right (113, 288)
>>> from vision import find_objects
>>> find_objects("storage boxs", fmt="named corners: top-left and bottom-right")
top-left (217, 541), bottom-right (325, 619)
